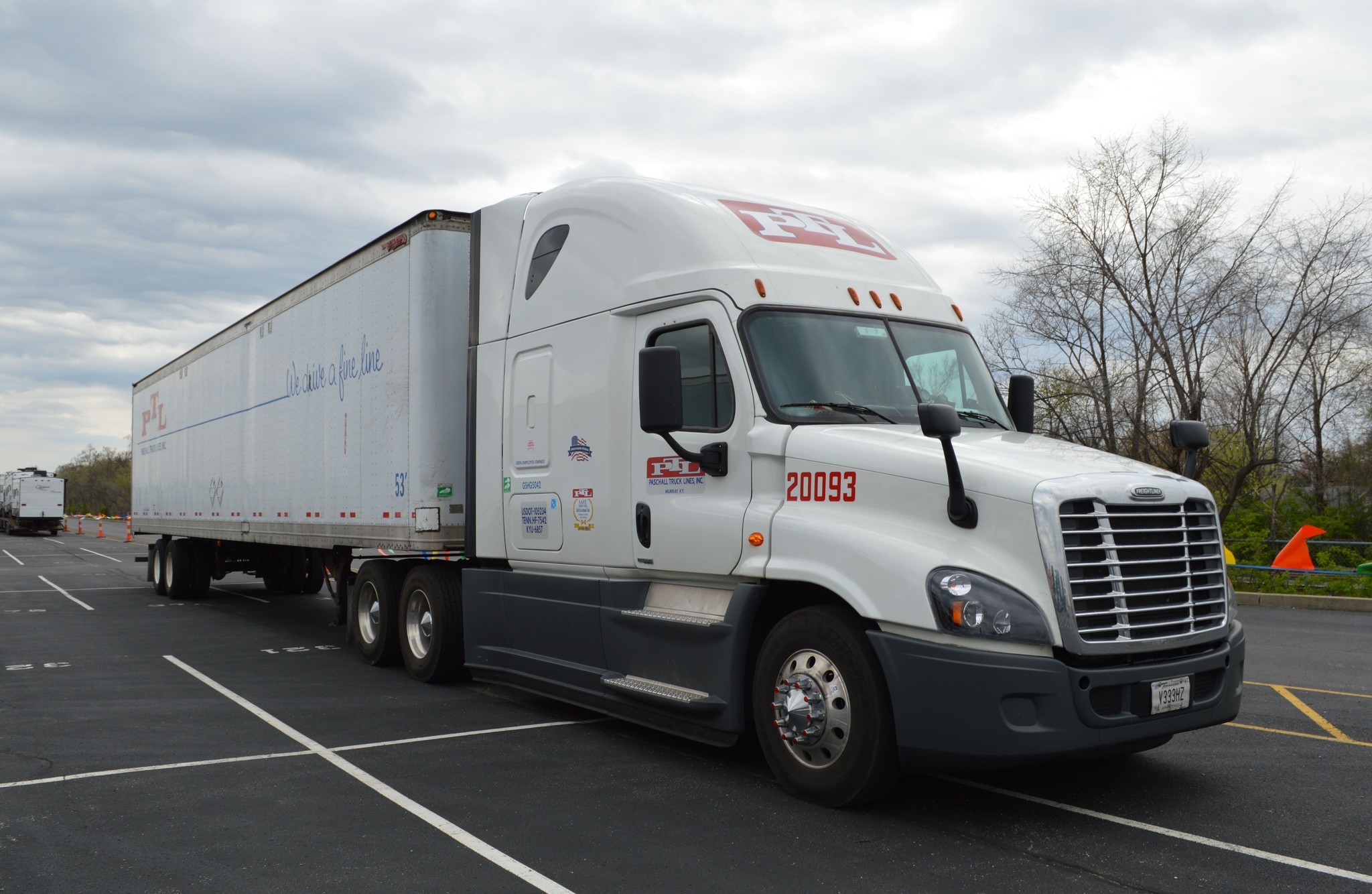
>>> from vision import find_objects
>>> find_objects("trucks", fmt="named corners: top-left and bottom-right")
top-left (0, 467), bottom-right (68, 535)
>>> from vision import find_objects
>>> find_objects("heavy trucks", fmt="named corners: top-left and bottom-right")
top-left (130, 176), bottom-right (1245, 811)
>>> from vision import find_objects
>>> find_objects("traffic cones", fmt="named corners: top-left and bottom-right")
top-left (96, 512), bottom-right (106, 538)
top-left (76, 512), bottom-right (84, 534)
top-left (61, 514), bottom-right (70, 532)
top-left (123, 513), bottom-right (134, 543)
